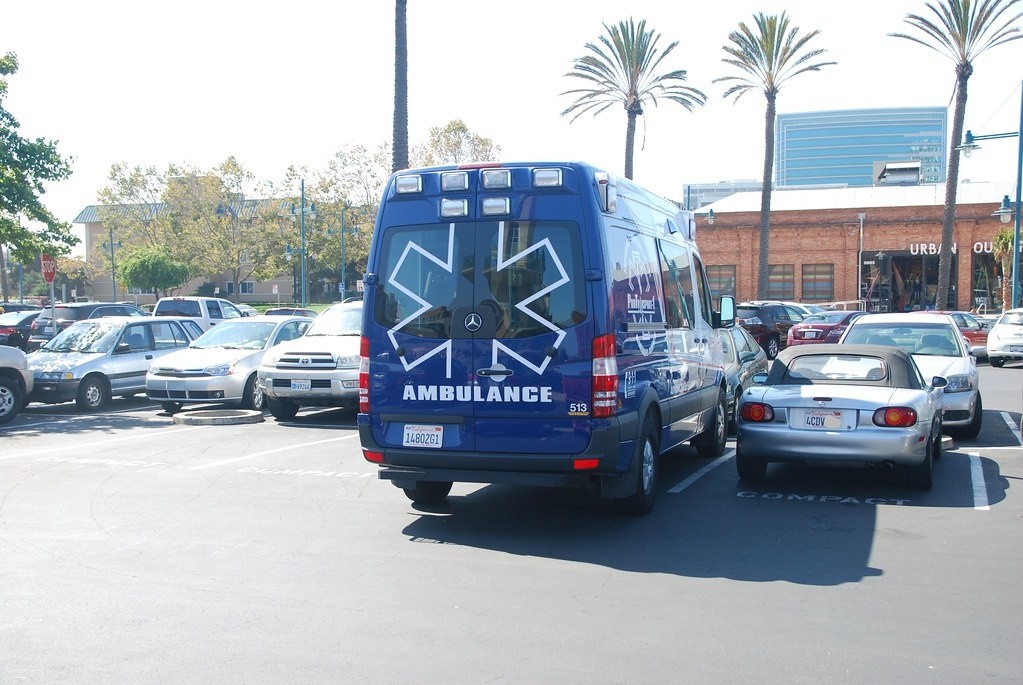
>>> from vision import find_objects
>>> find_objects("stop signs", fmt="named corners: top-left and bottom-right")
top-left (41, 250), bottom-right (57, 284)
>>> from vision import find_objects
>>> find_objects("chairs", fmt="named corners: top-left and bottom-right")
top-left (790, 368), bottom-right (884, 381)
top-left (866, 335), bottom-right (898, 347)
top-left (921, 335), bottom-right (956, 351)
top-left (130, 334), bottom-right (143, 348)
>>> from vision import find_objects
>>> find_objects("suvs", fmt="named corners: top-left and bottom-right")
top-left (255, 295), bottom-right (366, 422)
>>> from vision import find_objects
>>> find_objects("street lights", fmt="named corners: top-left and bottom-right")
top-left (947, 79), bottom-right (1023, 309)
top-left (101, 227), bottom-right (124, 302)
top-left (7, 216), bottom-right (26, 304)
top-left (875, 252), bottom-right (886, 313)
top-left (327, 204), bottom-right (365, 302)
top-left (657, 238), bottom-right (695, 331)
top-left (284, 177), bottom-right (317, 309)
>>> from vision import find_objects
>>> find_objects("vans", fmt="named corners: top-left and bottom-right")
top-left (356, 160), bottom-right (737, 517)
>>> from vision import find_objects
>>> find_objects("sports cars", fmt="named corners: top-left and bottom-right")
top-left (737, 343), bottom-right (950, 487)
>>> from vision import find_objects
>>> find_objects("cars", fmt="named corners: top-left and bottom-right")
top-left (0, 294), bottom-right (320, 352)
top-left (984, 308), bottom-right (1023, 367)
top-left (974, 289), bottom-right (987, 307)
top-left (0, 344), bottom-right (35, 425)
top-left (786, 310), bottom-right (884, 346)
top-left (910, 310), bottom-right (1000, 358)
top-left (143, 314), bottom-right (314, 414)
top-left (27, 316), bottom-right (207, 411)
top-left (820, 312), bottom-right (988, 440)
top-left (723, 299), bottom-right (826, 360)
